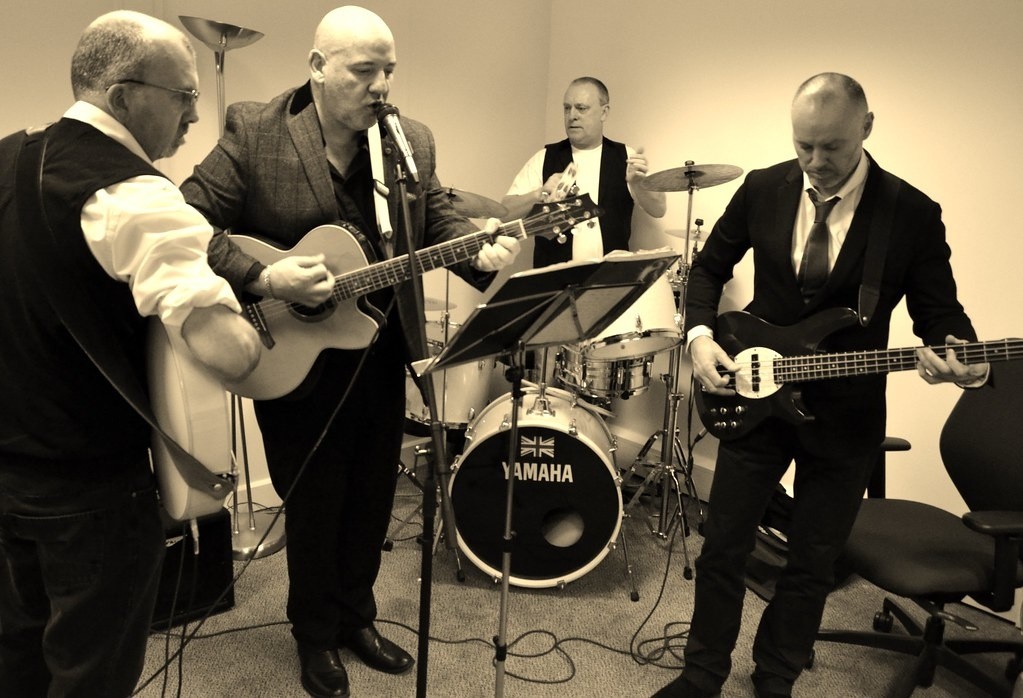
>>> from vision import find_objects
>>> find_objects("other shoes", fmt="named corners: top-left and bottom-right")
top-left (651, 676), bottom-right (721, 698)
top-left (754, 673), bottom-right (791, 698)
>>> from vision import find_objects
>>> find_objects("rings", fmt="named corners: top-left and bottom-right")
top-left (702, 386), bottom-right (707, 392)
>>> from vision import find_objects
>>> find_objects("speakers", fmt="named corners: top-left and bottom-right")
top-left (149, 505), bottom-right (234, 630)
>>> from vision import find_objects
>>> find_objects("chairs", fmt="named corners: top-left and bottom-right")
top-left (792, 340), bottom-right (1023, 698)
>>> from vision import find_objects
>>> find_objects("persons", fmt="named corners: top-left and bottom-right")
top-left (0, 9), bottom-right (262, 698)
top-left (500, 77), bottom-right (667, 269)
top-left (654, 72), bottom-right (991, 698)
top-left (180, 6), bottom-right (521, 698)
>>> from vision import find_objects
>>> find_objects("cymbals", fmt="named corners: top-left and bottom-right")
top-left (664, 228), bottom-right (711, 243)
top-left (440, 186), bottom-right (511, 221)
top-left (638, 163), bottom-right (745, 193)
top-left (546, 162), bottom-right (582, 203)
top-left (425, 298), bottom-right (458, 311)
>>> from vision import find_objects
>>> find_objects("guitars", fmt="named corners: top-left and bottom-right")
top-left (692, 311), bottom-right (1023, 441)
top-left (148, 315), bottom-right (232, 524)
top-left (224, 193), bottom-right (604, 401)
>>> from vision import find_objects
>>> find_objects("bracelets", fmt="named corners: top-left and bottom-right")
top-left (264, 265), bottom-right (276, 300)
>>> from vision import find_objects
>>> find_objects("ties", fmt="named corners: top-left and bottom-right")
top-left (798, 188), bottom-right (841, 289)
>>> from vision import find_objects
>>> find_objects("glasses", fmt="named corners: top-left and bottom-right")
top-left (105, 79), bottom-right (199, 103)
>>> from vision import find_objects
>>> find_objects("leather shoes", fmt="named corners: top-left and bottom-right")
top-left (298, 642), bottom-right (351, 698)
top-left (347, 625), bottom-right (415, 675)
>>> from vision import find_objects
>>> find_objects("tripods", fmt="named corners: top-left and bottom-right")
top-left (626, 177), bottom-right (704, 582)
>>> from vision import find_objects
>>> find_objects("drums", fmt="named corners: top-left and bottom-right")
top-left (404, 322), bottom-right (497, 430)
top-left (447, 385), bottom-right (624, 589)
top-left (556, 344), bottom-right (655, 399)
top-left (580, 253), bottom-right (688, 363)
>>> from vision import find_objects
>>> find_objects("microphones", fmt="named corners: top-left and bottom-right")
top-left (376, 102), bottom-right (419, 185)
